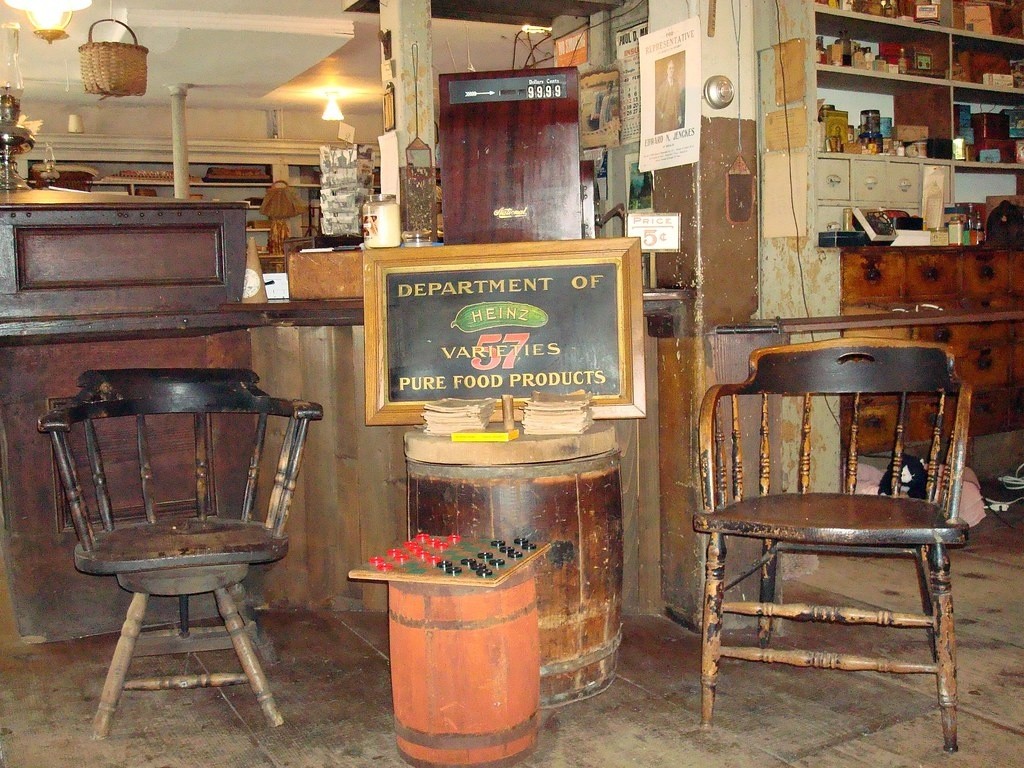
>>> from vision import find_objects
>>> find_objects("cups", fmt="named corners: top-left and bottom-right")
top-left (913, 142), bottom-right (927, 158)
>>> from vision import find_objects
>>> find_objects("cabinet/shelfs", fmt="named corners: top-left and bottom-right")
top-left (28, 156), bottom-right (324, 257)
top-left (751, 1), bottom-right (1024, 454)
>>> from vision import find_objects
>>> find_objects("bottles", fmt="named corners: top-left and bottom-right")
top-left (362, 194), bottom-right (401, 248)
top-left (847, 125), bottom-right (854, 144)
top-left (898, 49), bottom-right (908, 74)
top-left (884, 0), bottom-right (893, 18)
top-left (928, 202), bottom-right (985, 246)
top-left (923, 216), bottom-right (927, 231)
top-left (842, 208), bottom-right (852, 231)
top-left (896, 140), bottom-right (905, 157)
top-left (816, 30), bottom-right (888, 73)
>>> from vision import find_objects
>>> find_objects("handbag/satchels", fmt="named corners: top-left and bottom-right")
top-left (259, 180), bottom-right (307, 219)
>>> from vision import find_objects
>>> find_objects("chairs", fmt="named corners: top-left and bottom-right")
top-left (692, 335), bottom-right (974, 753)
top-left (36, 367), bottom-right (325, 744)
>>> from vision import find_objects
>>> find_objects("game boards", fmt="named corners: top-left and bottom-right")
top-left (348, 534), bottom-right (551, 580)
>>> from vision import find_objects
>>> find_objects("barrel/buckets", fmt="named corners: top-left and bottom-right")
top-left (388, 424), bottom-right (625, 768)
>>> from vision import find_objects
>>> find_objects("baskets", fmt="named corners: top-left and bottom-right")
top-left (78, 19), bottom-right (149, 96)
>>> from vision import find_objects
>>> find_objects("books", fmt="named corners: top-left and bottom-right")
top-left (519, 392), bottom-right (594, 434)
top-left (421, 398), bottom-right (494, 436)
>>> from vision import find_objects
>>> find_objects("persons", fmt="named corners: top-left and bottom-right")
top-left (655, 59), bottom-right (683, 134)
top-left (591, 81), bottom-right (615, 130)
